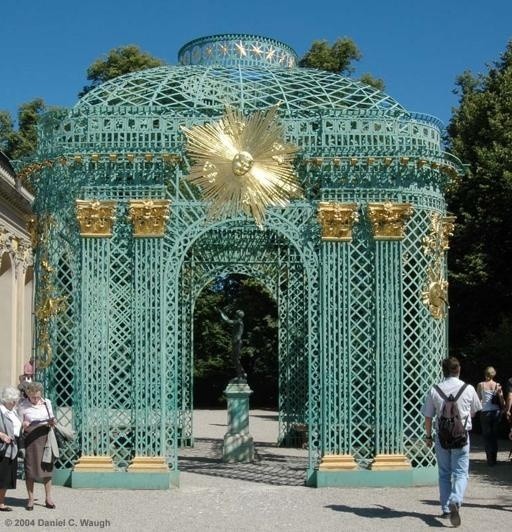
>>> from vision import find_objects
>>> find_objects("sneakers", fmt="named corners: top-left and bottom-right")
top-left (439, 501), bottom-right (462, 527)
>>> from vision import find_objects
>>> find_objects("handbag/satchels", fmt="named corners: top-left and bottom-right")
top-left (491, 383), bottom-right (502, 405)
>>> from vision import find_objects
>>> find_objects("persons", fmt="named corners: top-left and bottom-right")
top-left (218, 306), bottom-right (250, 383)
top-left (476, 365), bottom-right (506, 466)
top-left (505, 377), bottom-right (512, 461)
top-left (18, 382), bottom-right (56, 511)
top-left (423, 356), bottom-right (483, 526)
top-left (0, 386), bottom-right (22, 511)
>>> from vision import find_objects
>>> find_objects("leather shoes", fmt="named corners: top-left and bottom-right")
top-left (0, 499), bottom-right (56, 511)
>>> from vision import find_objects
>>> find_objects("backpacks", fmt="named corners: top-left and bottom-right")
top-left (432, 382), bottom-right (469, 449)
